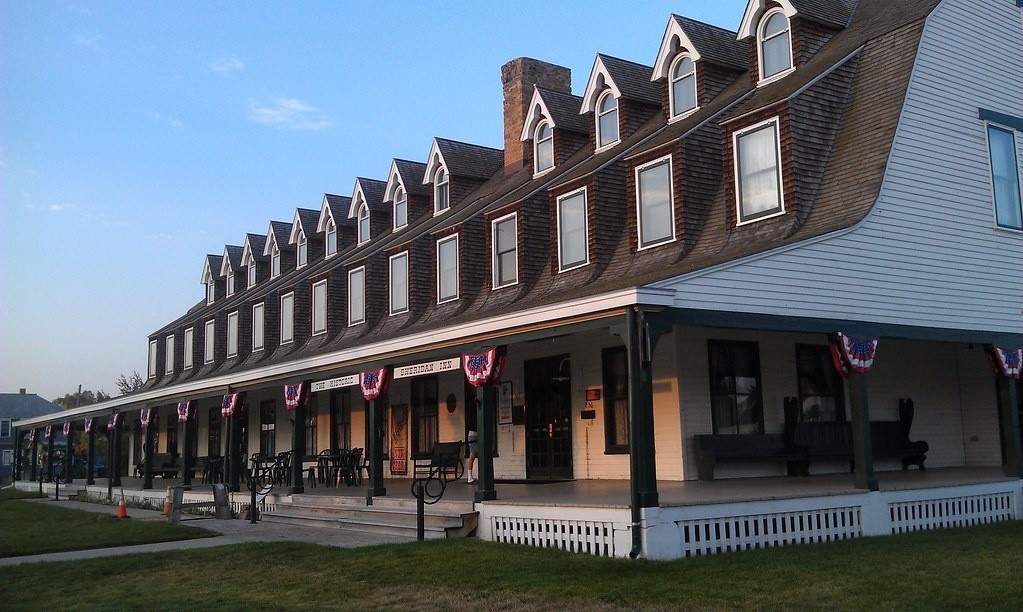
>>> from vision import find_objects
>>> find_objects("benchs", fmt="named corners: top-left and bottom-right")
top-left (694, 433), bottom-right (809, 482)
top-left (784, 394), bottom-right (929, 477)
top-left (138, 453), bottom-right (209, 479)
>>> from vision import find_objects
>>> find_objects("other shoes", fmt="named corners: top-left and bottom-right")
top-left (467, 480), bottom-right (477, 484)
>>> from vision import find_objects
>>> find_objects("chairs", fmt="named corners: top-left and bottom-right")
top-left (200, 454), bottom-right (248, 485)
top-left (248, 446), bottom-right (370, 488)
top-left (410, 440), bottom-right (462, 480)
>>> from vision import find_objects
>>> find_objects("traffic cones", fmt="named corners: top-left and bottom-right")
top-left (113, 486), bottom-right (131, 518)
top-left (161, 485), bottom-right (172, 515)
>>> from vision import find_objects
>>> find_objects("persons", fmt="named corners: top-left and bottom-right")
top-left (466, 399), bottom-right (478, 486)
